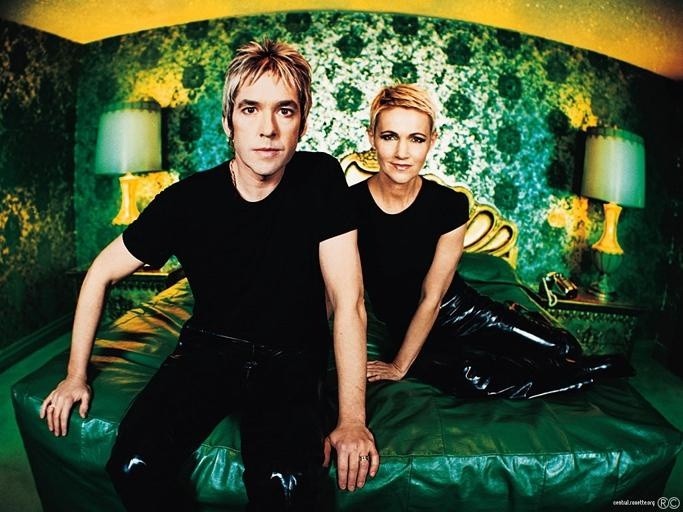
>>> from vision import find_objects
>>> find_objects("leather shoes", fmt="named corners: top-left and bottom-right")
top-left (586, 356), bottom-right (615, 366)
top-left (582, 359), bottom-right (638, 382)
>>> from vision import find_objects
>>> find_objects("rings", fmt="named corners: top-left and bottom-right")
top-left (48, 404), bottom-right (53, 408)
top-left (360, 456), bottom-right (368, 459)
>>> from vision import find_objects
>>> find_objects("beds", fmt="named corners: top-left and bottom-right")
top-left (12, 155), bottom-right (682, 512)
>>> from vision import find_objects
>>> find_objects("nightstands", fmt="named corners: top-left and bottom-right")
top-left (66, 261), bottom-right (184, 326)
top-left (534, 291), bottom-right (640, 383)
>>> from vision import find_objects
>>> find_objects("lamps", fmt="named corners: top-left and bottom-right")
top-left (581, 127), bottom-right (646, 301)
top-left (94, 101), bottom-right (163, 274)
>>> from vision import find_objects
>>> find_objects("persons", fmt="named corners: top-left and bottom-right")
top-left (324, 86), bottom-right (638, 401)
top-left (39, 36), bottom-right (380, 512)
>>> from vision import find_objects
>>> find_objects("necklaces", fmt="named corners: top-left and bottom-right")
top-left (231, 158), bottom-right (238, 186)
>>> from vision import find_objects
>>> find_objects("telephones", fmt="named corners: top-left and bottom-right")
top-left (540, 269), bottom-right (579, 307)
